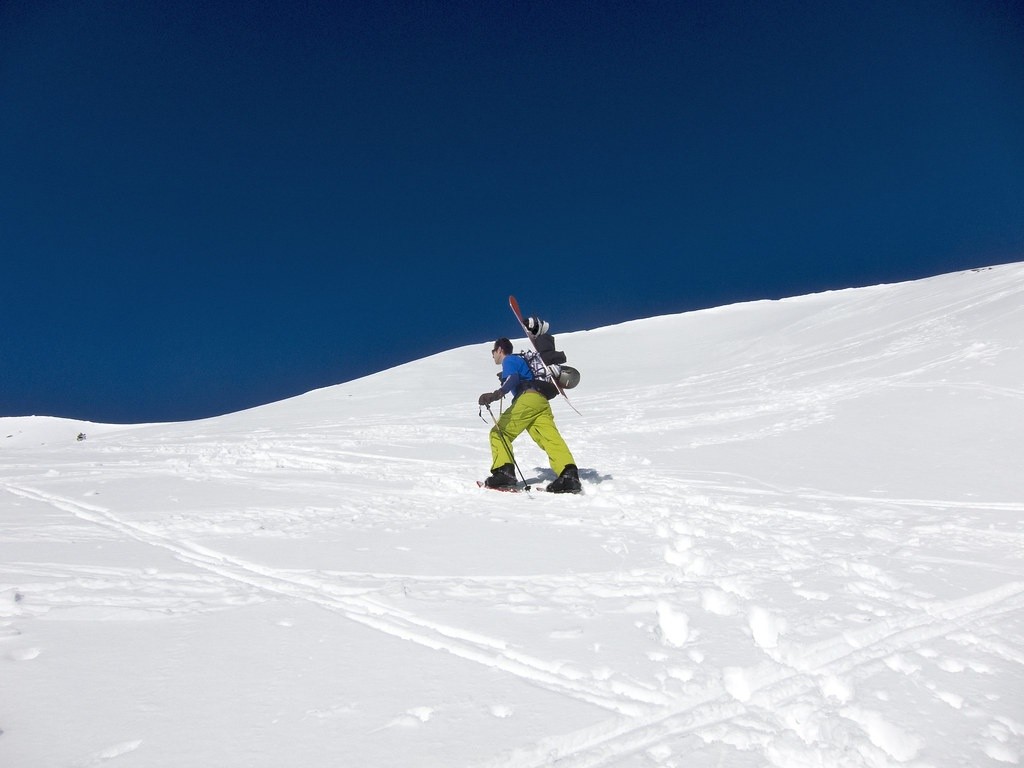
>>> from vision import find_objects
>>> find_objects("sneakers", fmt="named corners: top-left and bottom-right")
top-left (485, 463), bottom-right (517, 493)
top-left (546, 464), bottom-right (582, 494)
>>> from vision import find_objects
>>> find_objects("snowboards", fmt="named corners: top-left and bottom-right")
top-left (508, 294), bottom-right (583, 418)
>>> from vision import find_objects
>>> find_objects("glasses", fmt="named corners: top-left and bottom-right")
top-left (492, 348), bottom-right (499, 355)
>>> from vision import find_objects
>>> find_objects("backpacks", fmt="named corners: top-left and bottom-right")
top-left (511, 350), bottom-right (557, 401)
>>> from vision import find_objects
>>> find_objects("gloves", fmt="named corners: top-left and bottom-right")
top-left (478, 389), bottom-right (504, 405)
top-left (497, 372), bottom-right (503, 383)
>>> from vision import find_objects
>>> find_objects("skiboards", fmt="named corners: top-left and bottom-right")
top-left (475, 477), bottom-right (589, 498)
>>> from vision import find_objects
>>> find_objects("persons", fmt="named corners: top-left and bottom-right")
top-left (478, 337), bottom-right (582, 494)
